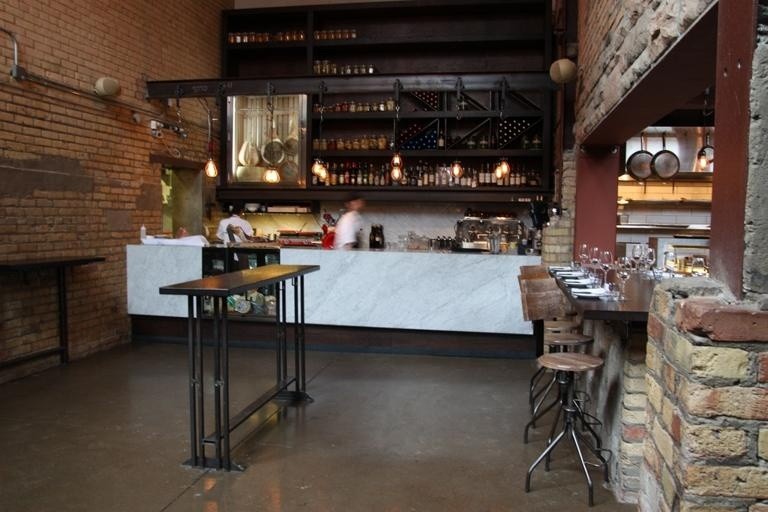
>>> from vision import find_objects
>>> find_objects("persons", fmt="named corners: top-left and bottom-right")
top-left (331, 190), bottom-right (370, 249)
top-left (216, 200), bottom-right (253, 270)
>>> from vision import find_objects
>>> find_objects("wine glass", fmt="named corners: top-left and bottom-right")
top-left (577, 241), bottom-right (631, 303)
top-left (631, 243), bottom-right (711, 284)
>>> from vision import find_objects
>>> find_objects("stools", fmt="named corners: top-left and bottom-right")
top-left (518, 257), bottom-right (614, 509)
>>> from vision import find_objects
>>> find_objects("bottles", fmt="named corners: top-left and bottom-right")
top-left (228, 27), bottom-right (357, 43)
top-left (520, 133), bottom-right (541, 149)
top-left (413, 90), bottom-right (438, 110)
top-left (394, 121), bottom-right (444, 151)
top-left (478, 158), bottom-right (543, 187)
top-left (446, 133), bottom-right (488, 150)
top-left (313, 133), bottom-right (395, 151)
top-left (368, 223), bottom-right (386, 249)
top-left (313, 60), bottom-right (377, 74)
top-left (313, 98), bottom-right (396, 113)
top-left (399, 160), bottom-right (478, 189)
top-left (310, 156), bottom-right (391, 186)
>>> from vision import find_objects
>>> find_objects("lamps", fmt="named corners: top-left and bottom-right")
top-left (205, 96), bottom-right (218, 178)
top-left (451, 78), bottom-right (463, 180)
top-left (262, 83), bottom-right (281, 186)
top-left (493, 73), bottom-right (510, 182)
top-left (390, 77), bottom-right (404, 182)
top-left (310, 80), bottom-right (330, 183)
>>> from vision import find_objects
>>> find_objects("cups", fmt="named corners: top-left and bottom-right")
top-left (617, 214), bottom-right (629, 224)
top-left (386, 231), bottom-right (455, 253)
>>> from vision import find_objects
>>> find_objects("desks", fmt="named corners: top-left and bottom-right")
top-left (1, 255), bottom-right (106, 373)
top-left (160, 262), bottom-right (320, 472)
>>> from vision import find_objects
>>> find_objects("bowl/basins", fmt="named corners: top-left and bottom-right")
top-left (245, 202), bottom-right (260, 213)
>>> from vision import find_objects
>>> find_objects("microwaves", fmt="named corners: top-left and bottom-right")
top-left (497, 118), bottom-right (531, 146)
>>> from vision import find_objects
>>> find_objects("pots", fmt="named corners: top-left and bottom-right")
top-left (650, 132), bottom-right (680, 181)
top-left (235, 112), bottom-right (301, 182)
top-left (627, 132), bottom-right (654, 183)
top-left (697, 132), bottom-right (715, 164)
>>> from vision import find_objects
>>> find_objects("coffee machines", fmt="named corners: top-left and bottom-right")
top-left (453, 214), bottom-right (523, 252)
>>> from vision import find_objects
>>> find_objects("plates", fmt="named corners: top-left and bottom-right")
top-left (549, 264), bottom-right (611, 298)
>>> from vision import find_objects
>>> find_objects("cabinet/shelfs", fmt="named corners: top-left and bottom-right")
top-left (213, 0), bottom-right (557, 201)
